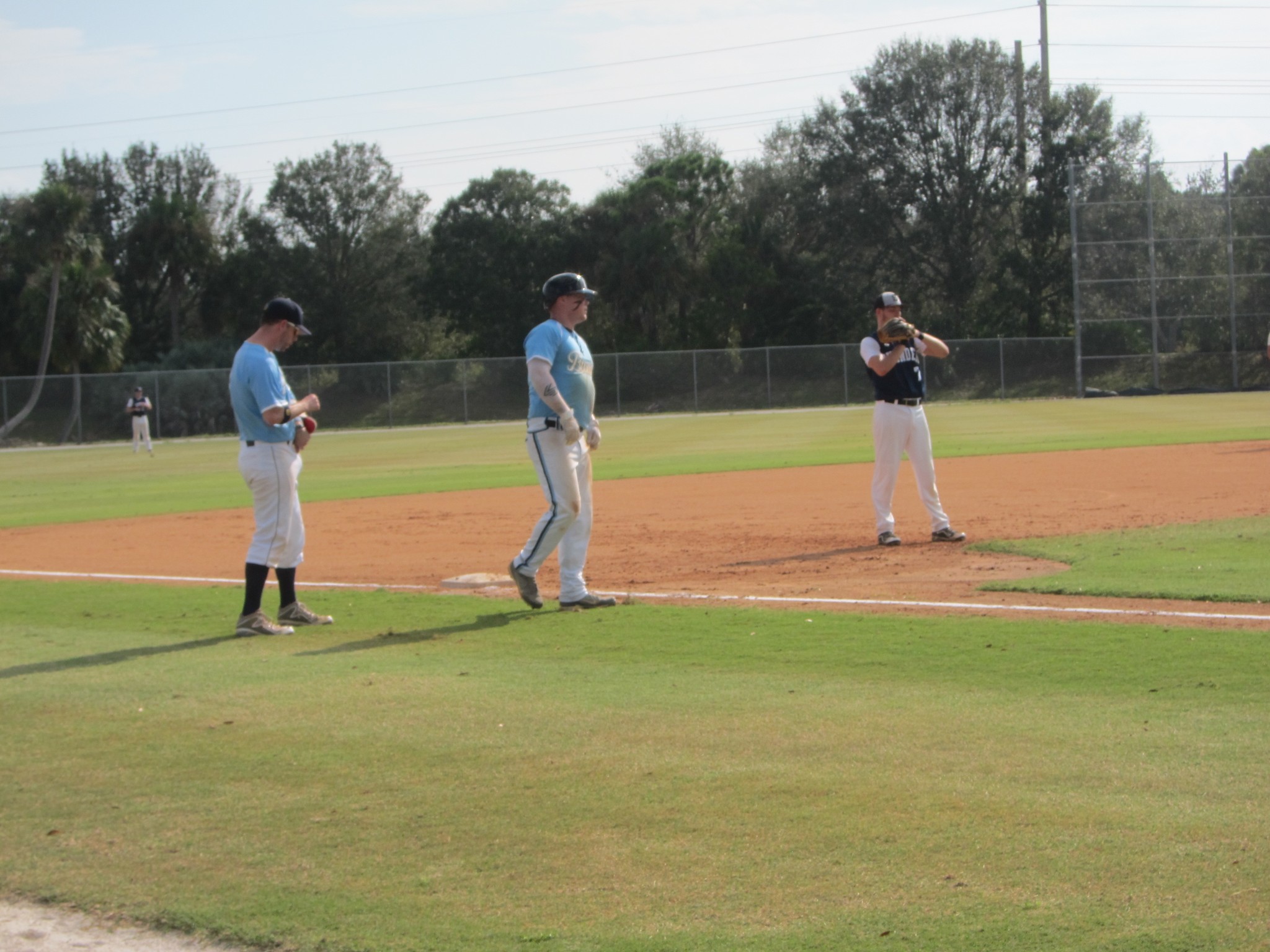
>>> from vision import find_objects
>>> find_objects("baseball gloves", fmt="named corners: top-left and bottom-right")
top-left (877, 317), bottom-right (918, 344)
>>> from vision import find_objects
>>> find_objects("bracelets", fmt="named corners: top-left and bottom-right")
top-left (919, 331), bottom-right (924, 340)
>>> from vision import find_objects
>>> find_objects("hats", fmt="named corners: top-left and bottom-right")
top-left (264, 297), bottom-right (313, 337)
top-left (135, 387), bottom-right (142, 392)
top-left (874, 292), bottom-right (903, 308)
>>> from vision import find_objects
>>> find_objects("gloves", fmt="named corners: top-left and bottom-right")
top-left (586, 420), bottom-right (600, 450)
top-left (558, 408), bottom-right (580, 446)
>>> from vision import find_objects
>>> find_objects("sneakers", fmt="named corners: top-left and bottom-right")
top-left (236, 612), bottom-right (294, 637)
top-left (931, 527), bottom-right (966, 542)
top-left (508, 561), bottom-right (543, 610)
top-left (558, 593), bottom-right (617, 611)
top-left (879, 531), bottom-right (901, 546)
top-left (275, 600), bottom-right (332, 627)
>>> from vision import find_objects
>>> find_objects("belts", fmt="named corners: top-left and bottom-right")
top-left (885, 398), bottom-right (921, 406)
top-left (545, 420), bottom-right (584, 433)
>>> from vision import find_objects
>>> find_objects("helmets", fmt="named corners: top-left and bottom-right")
top-left (540, 272), bottom-right (598, 309)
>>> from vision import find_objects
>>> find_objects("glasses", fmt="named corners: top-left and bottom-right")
top-left (286, 320), bottom-right (298, 335)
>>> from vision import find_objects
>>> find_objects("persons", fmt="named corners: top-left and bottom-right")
top-left (860, 292), bottom-right (966, 545)
top-left (508, 273), bottom-right (616, 609)
top-left (126, 386), bottom-right (155, 457)
top-left (229, 298), bottom-right (333, 636)
